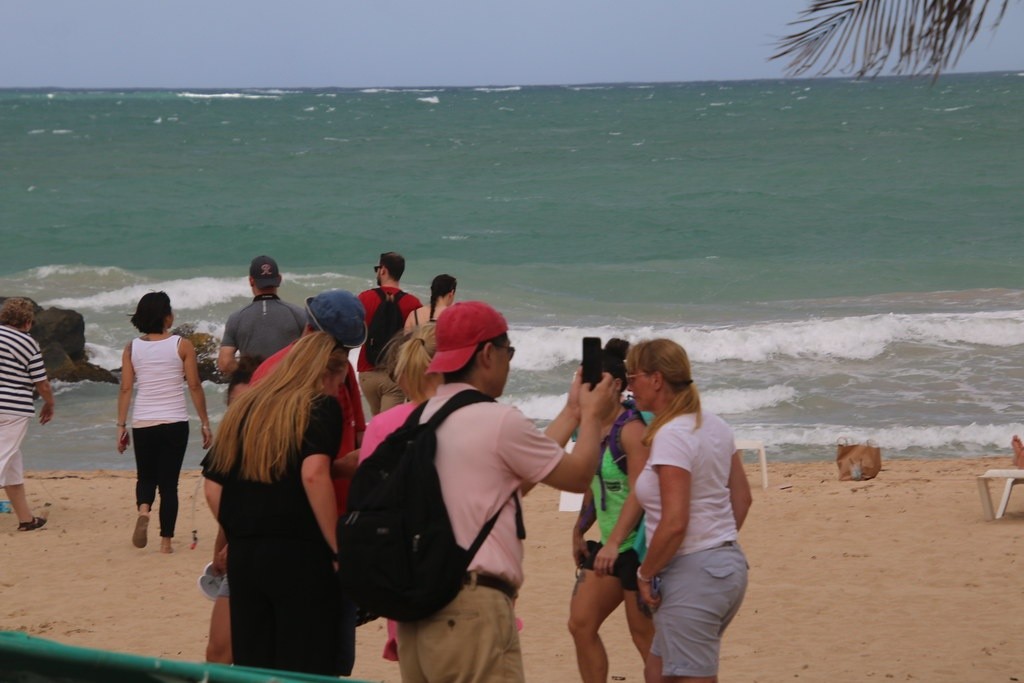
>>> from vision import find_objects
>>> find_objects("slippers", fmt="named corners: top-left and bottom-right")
top-left (132, 515), bottom-right (149, 549)
top-left (159, 547), bottom-right (173, 553)
top-left (197, 561), bottom-right (229, 602)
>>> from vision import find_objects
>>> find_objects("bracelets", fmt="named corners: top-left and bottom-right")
top-left (203, 426), bottom-right (209, 429)
top-left (637, 566), bottom-right (650, 583)
top-left (117, 423), bottom-right (126, 427)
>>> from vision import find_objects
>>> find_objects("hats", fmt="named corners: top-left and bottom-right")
top-left (249, 255), bottom-right (279, 289)
top-left (425, 301), bottom-right (508, 374)
top-left (304, 290), bottom-right (369, 349)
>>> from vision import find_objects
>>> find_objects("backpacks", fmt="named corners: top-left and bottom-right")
top-left (365, 288), bottom-right (407, 363)
top-left (334, 389), bottom-right (516, 625)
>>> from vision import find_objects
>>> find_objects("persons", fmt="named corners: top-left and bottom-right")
top-left (625, 338), bottom-right (752, 683)
top-left (0, 297), bottom-right (54, 531)
top-left (217, 255), bottom-right (307, 373)
top-left (404, 274), bottom-right (458, 335)
top-left (1011, 435), bottom-right (1024, 469)
top-left (397, 302), bottom-right (618, 683)
top-left (358, 322), bottom-right (523, 660)
top-left (567, 339), bottom-right (655, 683)
top-left (200, 289), bottom-right (368, 679)
top-left (357, 252), bottom-right (423, 416)
top-left (117, 291), bottom-right (213, 554)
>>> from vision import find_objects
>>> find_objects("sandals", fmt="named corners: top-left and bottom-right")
top-left (17, 517), bottom-right (45, 531)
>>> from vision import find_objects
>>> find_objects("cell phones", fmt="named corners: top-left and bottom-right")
top-left (582, 337), bottom-right (602, 392)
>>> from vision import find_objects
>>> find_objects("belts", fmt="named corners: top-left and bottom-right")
top-left (464, 572), bottom-right (516, 598)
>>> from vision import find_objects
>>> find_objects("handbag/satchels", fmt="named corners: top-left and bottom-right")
top-left (834, 435), bottom-right (881, 481)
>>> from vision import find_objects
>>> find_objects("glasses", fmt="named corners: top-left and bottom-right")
top-left (374, 265), bottom-right (381, 272)
top-left (625, 372), bottom-right (647, 386)
top-left (479, 345), bottom-right (514, 361)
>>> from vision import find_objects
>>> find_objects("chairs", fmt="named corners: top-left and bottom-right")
top-left (977, 469), bottom-right (1024, 521)
top-left (733, 440), bottom-right (769, 486)
